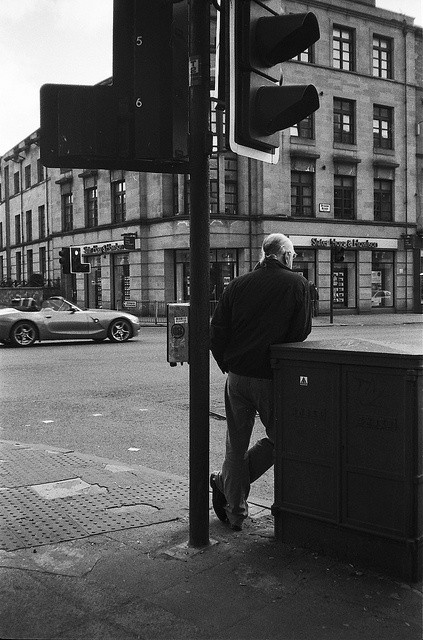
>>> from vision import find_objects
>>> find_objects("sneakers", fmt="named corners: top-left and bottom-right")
top-left (210, 471), bottom-right (227, 522)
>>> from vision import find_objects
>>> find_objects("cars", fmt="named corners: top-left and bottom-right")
top-left (0, 296), bottom-right (139, 348)
top-left (371, 291), bottom-right (392, 307)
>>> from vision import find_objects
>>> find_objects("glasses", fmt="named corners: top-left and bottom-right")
top-left (288, 251), bottom-right (297, 258)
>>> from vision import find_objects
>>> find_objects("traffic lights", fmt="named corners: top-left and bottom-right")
top-left (227, 0), bottom-right (318, 163)
top-left (59, 246), bottom-right (70, 274)
top-left (335, 246), bottom-right (345, 262)
top-left (70, 246), bottom-right (81, 273)
top-left (81, 263), bottom-right (91, 274)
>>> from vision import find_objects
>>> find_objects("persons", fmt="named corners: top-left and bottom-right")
top-left (210, 233), bottom-right (315, 530)
